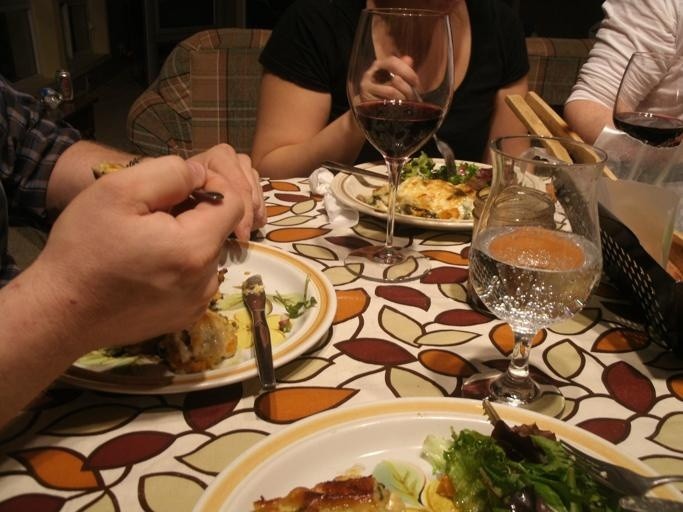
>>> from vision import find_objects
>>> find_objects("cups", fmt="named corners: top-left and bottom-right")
top-left (464, 185), bottom-right (558, 318)
top-left (610, 48), bottom-right (682, 144)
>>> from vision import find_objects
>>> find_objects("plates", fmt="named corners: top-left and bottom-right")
top-left (325, 154), bottom-right (545, 232)
top-left (48, 233), bottom-right (340, 397)
top-left (187, 393), bottom-right (683, 511)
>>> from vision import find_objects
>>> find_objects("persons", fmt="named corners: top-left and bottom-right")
top-left (248, 0), bottom-right (537, 176)
top-left (1, 71), bottom-right (267, 426)
top-left (564, 0), bottom-right (683, 168)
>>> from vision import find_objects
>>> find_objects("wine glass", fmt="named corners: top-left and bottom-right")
top-left (459, 130), bottom-right (605, 419)
top-left (342, 5), bottom-right (456, 283)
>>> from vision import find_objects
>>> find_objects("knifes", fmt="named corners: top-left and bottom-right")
top-left (239, 272), bottom-right (278, 389)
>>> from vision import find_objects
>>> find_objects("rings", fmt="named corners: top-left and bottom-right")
top-left (373, 68), bottom-right (395, 85)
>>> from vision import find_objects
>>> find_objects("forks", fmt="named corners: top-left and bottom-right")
top-left (558, 436), bottom-right (683, 497)
top-left (389, 73), bottom-right (459, 175)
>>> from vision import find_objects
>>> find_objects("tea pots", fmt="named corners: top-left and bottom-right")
top-left (38, 87), bottom-right (63, 110)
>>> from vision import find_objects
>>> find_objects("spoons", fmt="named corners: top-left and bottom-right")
top-left (90, 163), bottom-right (225, 201)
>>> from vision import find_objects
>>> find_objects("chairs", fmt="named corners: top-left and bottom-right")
top-left (124, 29), bottom-right (274, 167)
top-left (522, 36), bottom-right (597, 109)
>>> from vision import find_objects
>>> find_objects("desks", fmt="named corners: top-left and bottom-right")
top-left (41, 93), bottom-right (101, 141)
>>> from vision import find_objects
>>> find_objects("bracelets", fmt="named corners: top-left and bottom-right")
top-left (125, 151), bottom-right (145, 167)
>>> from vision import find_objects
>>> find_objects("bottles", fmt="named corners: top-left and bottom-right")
top-left (56, 70), bottom-right (74, 101)
